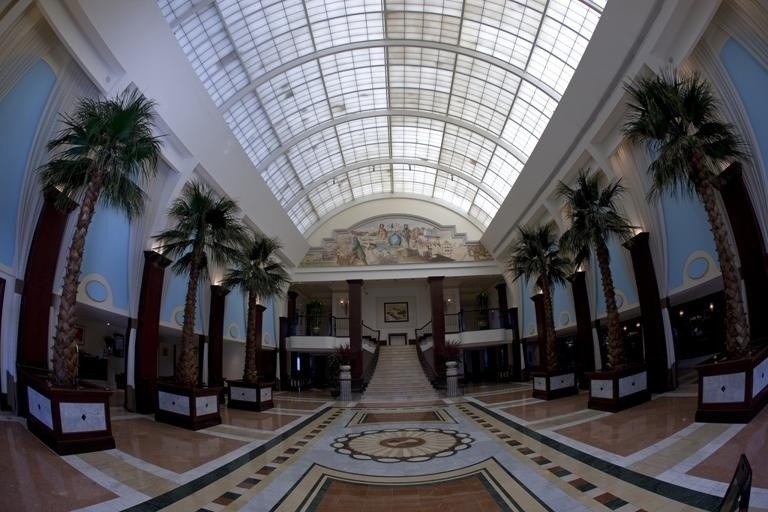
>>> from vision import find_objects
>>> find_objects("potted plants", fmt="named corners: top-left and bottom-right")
top-left (505, 219), bottom-right (579, 401)
top-left (217, 233), bottom-right (293, 414)
top-left (151, 175), bottom-right (254, 431)
top-left (553, 167), bottom-right (653, 412)
top-left (15, 84), bottom-right (169, 460)
top-left (329, 342), bottom-right (354, 396)
top-left (475, 285), bottom-right (491, 329)
top-left (618, 61), bottom-right (768, 425)
top-left (305, 293), bottom-right (327, 336)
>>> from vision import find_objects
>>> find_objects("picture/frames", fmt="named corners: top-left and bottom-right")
top-left (383, 301), bottom-right (410, 323)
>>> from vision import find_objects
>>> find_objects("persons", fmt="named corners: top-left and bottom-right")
top-left (349, 223), bottom-right (443, 266)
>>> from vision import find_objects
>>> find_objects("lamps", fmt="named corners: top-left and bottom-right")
top-left (442, 294), bottom-right (455, 315)
top-left (336, 295), bottom-right (349, 317)
